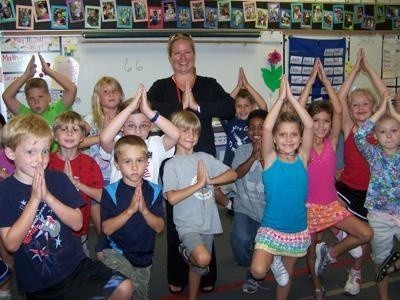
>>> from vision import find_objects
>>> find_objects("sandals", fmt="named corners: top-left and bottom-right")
top-left (169, 282), bottom-right (184, 294)
top-left (201, 286), bottom-right (214, 292)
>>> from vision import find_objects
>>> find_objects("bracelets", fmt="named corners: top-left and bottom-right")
top-left (151, 109), bottom-right (159, 123)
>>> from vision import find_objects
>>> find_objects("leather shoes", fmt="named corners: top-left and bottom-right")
top-left (373, 248), bottom-right (400, 283)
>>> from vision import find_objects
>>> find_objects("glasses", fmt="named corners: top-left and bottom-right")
top-left (168, 32), bottom-right (191, 44)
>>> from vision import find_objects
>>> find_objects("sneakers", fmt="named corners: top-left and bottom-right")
top-left (342, 266), bottom-right (363, 297)
top-left (313, 286), bottom-right (327, 300)
top-left (177, 242), bottom-right (211, 275)
top-left (315, 241), bottom-right (337, 279)
top-left (333, 228), bottom-right (364, 260)
top-left (241, 273), bottom-right (270, 296)
top-left (271, 255), bottom-right (290, 287)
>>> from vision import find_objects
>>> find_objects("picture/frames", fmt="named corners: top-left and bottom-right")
top-left (291, 3), bottom-right (304, 23)
top-left (321, 10), bottom-right (334, 31)
top-left (1, 0), bottom-right (17, 23)
top-left (267, 3), bottom-right (282, 23)
top-left (300, 9), bottom-right (313, 29)
top-left (49, 5), bottom-right (69, 30)
top-left (311, 3), bottom-right (325, 24)
top-left (131, 1), bottom-right (149, 22)
top-left (255, 7), bottom-right (269, 29)
top-left (202, 7), bottom-right (219, 29)
top-left (175, 6), bottom-right (192, 28)
top-left (31, 1), bottom-right (52, 22)
top-left (65, 1), bottom-right (87, 24)
top-left (99, 1), bottom-right (118, 23)
top-left (161, 1), bottom-right (178, 22)
top-left (15, 4), bottom-right (35, 30)
top-left (115, 5), bottom-right (134, 29)
top-left (242, 2), bottom-right (258, 23)
top-left (353, 4), bottom-right (365, 25)
top-left (361, 14), bottom-right (376, 31)
top-left (190, 1), bottom-right (207, 22)
top-left (332, 4), bottom-right (345, 24)
top-left (374, 1), bottom-right (386, 24)
top-left (279, 8), bottom-right (293, 28)
top-left (343, 10), bottom-right (354, 31)
top-left (84, 5), bottom-right (102, 29)
top-left (391, 16), bottom-right (400, 32)
top-left (146, 6), bottom-right (164, 29)
top-left (230, 7), bottom-right (245, 29)
top-left (217, 2), bottom-right (232, 22)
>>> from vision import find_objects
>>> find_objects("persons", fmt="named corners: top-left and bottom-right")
top-left (216, 66), bottom-right (268, 218)
top-left (79, 76), bottom-right (125, 239)
top-left (148, 31), bottom-right (236, 294)
top-left (329, 47), bottom-right (390, 294)
top-left (96, 136), bottom-right (166, 300)
top-left (354, 91), bottom-right (399, 300)
top-left (248, 74), bottom-right (317, 300)
top-left (0, 112), bottom-right (134, 300)
top-left (46, 112), bottom-right (104, 261)
top-left (230, 108), bottom-right (274, 295)
top-left (100, 83), bottom-right (180, 193)
top-left (163, 110), bottom-right (237, 300)
top-left (1, 1), bottom-right (399, 28)
top-left (0, 112), bottom-right (24, 299)
top-left (2, 52), bottom-right (77, 152)
top-left (295, 55), bottom-right (374, 299)
